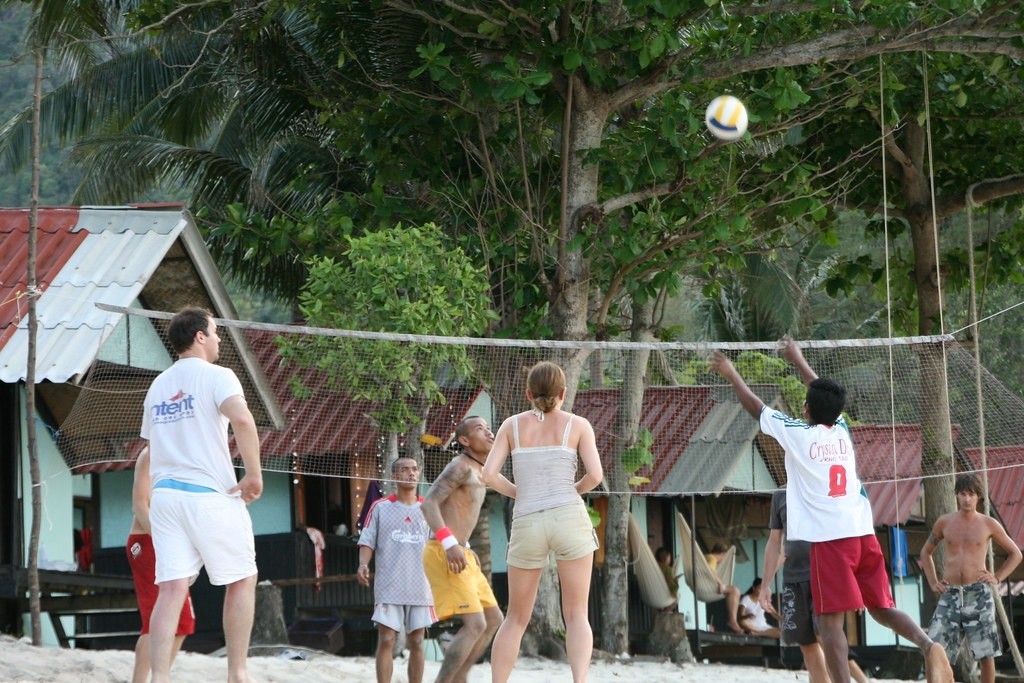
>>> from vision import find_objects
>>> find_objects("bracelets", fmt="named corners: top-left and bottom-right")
top-left (441, 536), bottom-right (458, 550)
top-left (995, 577), bottom-right (999, 585)
top-left (359, 565), bottom-right (367, 567)
top-left (435, 527), bottom-right (451, 542)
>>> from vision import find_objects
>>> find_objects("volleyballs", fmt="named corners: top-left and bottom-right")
top-left (703, 94), bottom-right (749, 141)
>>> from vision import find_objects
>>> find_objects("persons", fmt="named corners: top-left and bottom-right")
top-left (703, 544), bottom-right (744, 634)
top-left (125, 446), bottom-right (196, 683)
top-left (483, 362), bottom-right (603, 683)
top-left (740, 578), bottom-right (782, 637)
top-left (656, 547), bottom-right (681, 613)
top-left (708, 334), bottom-right (956, 683)
top-left (420, 416), bottom-right (504, 682)
top-left (357, 457), bottom-right (438, 683)
top-left (140, 307), bottom-right (263, 683)
top-left (921, 474), bottom-right (1023, 683)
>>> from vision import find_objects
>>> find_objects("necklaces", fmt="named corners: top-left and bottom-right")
top-left (463, 452), bottom-right (484, 466)
